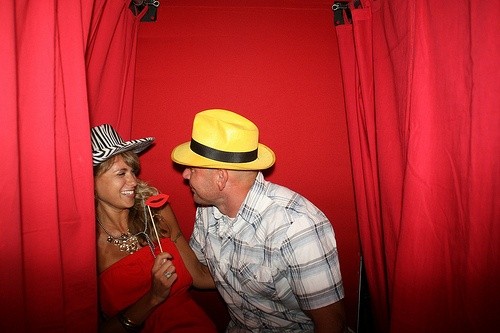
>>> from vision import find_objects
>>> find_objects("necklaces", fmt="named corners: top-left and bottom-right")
top-left (97, 216), bottom-right (139, 254)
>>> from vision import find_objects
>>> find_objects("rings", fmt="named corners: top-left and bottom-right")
top-left (164, 272), bottom-right (171, 278)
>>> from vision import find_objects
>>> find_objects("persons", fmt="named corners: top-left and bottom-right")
top-left (157, 109), bottom-right (348, 333)
top-left (90, 124), bottom-right (219, 333)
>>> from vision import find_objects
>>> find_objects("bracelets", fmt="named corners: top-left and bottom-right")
top-left (115, 312), bottom-right (142, 333)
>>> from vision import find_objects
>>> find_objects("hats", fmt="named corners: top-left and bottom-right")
top-left (171, 109), bottom-right (275, 170)
top-left (91, 124), bottom-right (155, 166)
